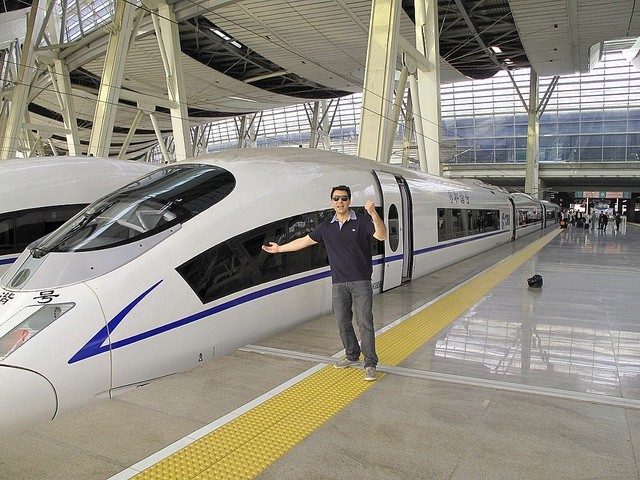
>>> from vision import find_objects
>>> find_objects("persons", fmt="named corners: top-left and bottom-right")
top-left (261, 185), bottom-right (388, 382)
top-left (559, 208), bottom-right (621, 231)
top-left (475, 213), bottom-right (500, 233)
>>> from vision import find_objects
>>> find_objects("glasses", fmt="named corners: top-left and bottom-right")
top-left (332, 195), bottom-right (349, 201)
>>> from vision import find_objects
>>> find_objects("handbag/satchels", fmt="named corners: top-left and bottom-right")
top-left (527, 275), bottom-right (543, 288)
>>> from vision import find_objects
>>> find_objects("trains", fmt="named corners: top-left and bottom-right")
top-left (0, 157), bottom-right (165, 278)
top-left (1, 148), bottom-right (562, 424)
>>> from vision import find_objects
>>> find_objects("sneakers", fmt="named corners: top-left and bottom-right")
top-left (334, 358), bottom-right (360, 368)
top-left (365, 367), bottom-right (376, 380)
